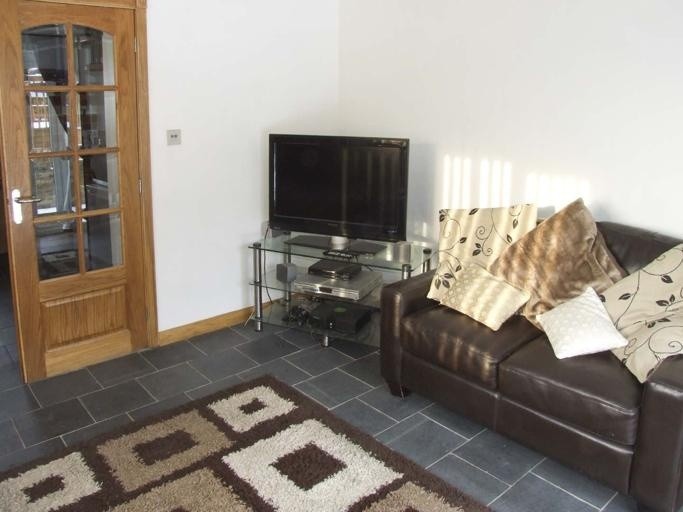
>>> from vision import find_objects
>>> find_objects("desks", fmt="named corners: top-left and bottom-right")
top-left (247, 232), bottom-right (437, 347)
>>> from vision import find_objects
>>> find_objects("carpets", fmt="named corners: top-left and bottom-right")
top-left (0, 373), bottom-right (495, 512)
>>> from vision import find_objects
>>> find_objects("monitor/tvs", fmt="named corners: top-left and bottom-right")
top-left (268, 133), bottom-right (409, 259)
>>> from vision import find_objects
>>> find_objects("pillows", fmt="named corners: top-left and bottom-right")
top-left (439, 260), bottom-right (532, 333)
top-left (484, 198), bottom-right (628, 331)
top-left (535, 285), bottom-right (629, 360)
top-left (597, 241), bottom-right (683, 384)
top-left (426, 203), bottom-right (539, 317)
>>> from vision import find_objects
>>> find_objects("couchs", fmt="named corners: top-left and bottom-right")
top-left (379, 217), bottom-right (683, 512)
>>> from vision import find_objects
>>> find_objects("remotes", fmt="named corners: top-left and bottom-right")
top-left (323, 250), bottom-right (359, 258)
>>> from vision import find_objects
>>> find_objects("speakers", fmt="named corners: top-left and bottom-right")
top-left (276, 264), bottom-right (296, 283)
